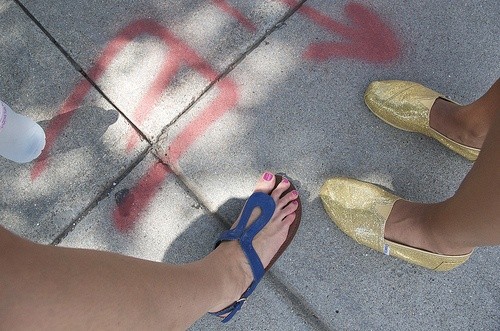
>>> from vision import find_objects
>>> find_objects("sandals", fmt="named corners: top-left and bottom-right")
top-left (205, 171), bottom-right (302, 322)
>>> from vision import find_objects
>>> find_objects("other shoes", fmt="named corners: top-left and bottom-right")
top-left (317, 177), bottom-right (475, 272)
top-left (364, 79), bottom-right (479, 161)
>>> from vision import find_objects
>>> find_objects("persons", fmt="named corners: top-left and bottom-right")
top-left (0, 171), bottom-right (302, 331)
top-left (318, 77), bottom-right (500, 271)
